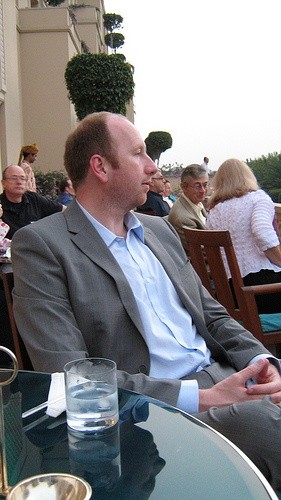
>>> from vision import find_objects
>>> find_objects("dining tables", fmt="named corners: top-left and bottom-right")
top-left (0, 369), bottom-right (280, 500)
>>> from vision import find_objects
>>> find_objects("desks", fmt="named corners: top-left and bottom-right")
top-left (0, 260), bottom-right (16, 279)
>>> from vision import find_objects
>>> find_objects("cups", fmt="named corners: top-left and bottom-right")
top-left (64, 357), bottom-right (119, 432)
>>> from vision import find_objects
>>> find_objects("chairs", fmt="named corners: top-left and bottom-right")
top-left (272, 205), bottom-right (281, 240)
top-left (0, 264), bottom-right (29, 370)
top-left (181, 225), bottom-right (281, 350)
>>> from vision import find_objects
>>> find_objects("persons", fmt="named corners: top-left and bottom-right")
top-left (168, 164), bottom-right (208, 262)
top-left (133, 171), bottom-right (174, 217)
top-left (17, 374), bottom-right (166, 500)
top-left (0, 165), bottom-right (67, 241)
top-left (10, 111), bottom-right (281, 492)
top-left (205, 159), bottom-right (281, 314)
top-left (201, 157), bottom-right (212, 175)
top-left (18, 143), bottom-right (39, 193)
top-left (55, 179), bottom-right (75, 206)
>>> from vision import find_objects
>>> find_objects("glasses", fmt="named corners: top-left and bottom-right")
top-left (151, 176), bottom-right (163, 179)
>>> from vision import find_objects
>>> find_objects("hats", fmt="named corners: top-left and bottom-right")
top-left (16, 143), bottom-right (38, 166)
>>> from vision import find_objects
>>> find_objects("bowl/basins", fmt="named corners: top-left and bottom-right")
top-left (7, 473), bottom-right (92, 500)
